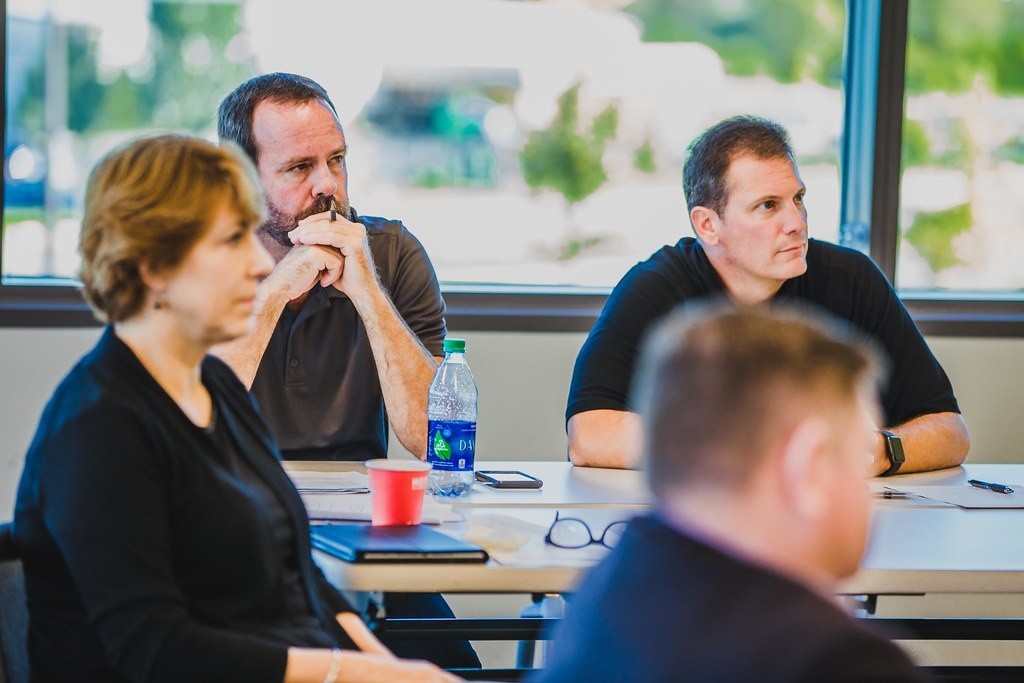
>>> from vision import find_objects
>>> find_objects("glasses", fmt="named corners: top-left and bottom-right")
top-left (546, 509), bottom-right (633, 550)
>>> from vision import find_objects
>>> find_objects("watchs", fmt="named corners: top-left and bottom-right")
top-left (873, 430), bottom-right (905, 477)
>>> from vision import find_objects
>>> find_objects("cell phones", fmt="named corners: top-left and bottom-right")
top-left (475, 470), bottom-right (543, 488)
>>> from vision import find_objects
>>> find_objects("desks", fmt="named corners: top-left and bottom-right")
top-left (276, 460), bottom-right (1024, 683)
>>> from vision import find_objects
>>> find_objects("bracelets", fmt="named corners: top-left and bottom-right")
top-left (323, 648), bottom-right (342, 683)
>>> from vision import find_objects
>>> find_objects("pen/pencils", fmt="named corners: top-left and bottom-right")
top-left (873, 491), bottom-right (913, 500)
top-left (330, 198), bottom-right (337, 221)
top-left (967, 479), bottom-right (1015, 494)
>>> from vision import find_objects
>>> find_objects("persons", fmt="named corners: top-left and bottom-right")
top-left (11, 134), bottom-right (466, 683)
top-left (532, 313), bottom-right (925, 683)
top-left (566, 115), bottom-right (971, 474)
top-left (207, 73), bottom-right (485, 670)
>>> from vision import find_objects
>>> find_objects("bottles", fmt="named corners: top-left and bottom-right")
top-left (426, 338), bottom-right (479, 496)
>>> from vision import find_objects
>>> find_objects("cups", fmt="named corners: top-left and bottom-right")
top-left (365, 459), bottom-right (433, 526)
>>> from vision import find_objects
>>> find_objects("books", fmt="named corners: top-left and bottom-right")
top-left (308, 524), bottom-right (489, 564)
